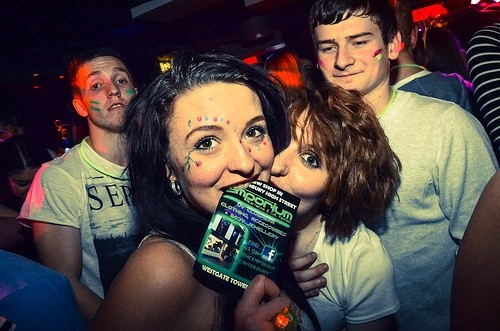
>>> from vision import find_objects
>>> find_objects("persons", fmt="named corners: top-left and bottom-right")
top-left (0, 113), bottom-right (86, 264)
top-left (424, 27), bottom-right (471, 82)
top-left (16, 48), bottom-right (138, 325)
top-left (269, 81), bottom-right (402, 331)
top-left (389, 6), bottom-right (475, 117)
top-left (0, 249), bottom-right (85, 331)
top-left (466, 22), bottom-right (500, 162)
top-left (85, 53), bottom-right (302, 331)
top-left (264, 53), bottom-right (325, 94)
top-left (308, 0), bottom-right (500, 331)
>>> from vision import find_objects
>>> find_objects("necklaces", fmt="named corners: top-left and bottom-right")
top-left (389, 64), bottom-right (426, 70)
top-left (377, 86), bottom-right (396, 119)
top-left (80, 136), bottom-right (131, 180)
top-left (304, 226), bottom-right (321, 254)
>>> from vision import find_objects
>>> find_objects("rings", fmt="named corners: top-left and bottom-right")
top-left (272, 305), bottom-right (302, 331)
